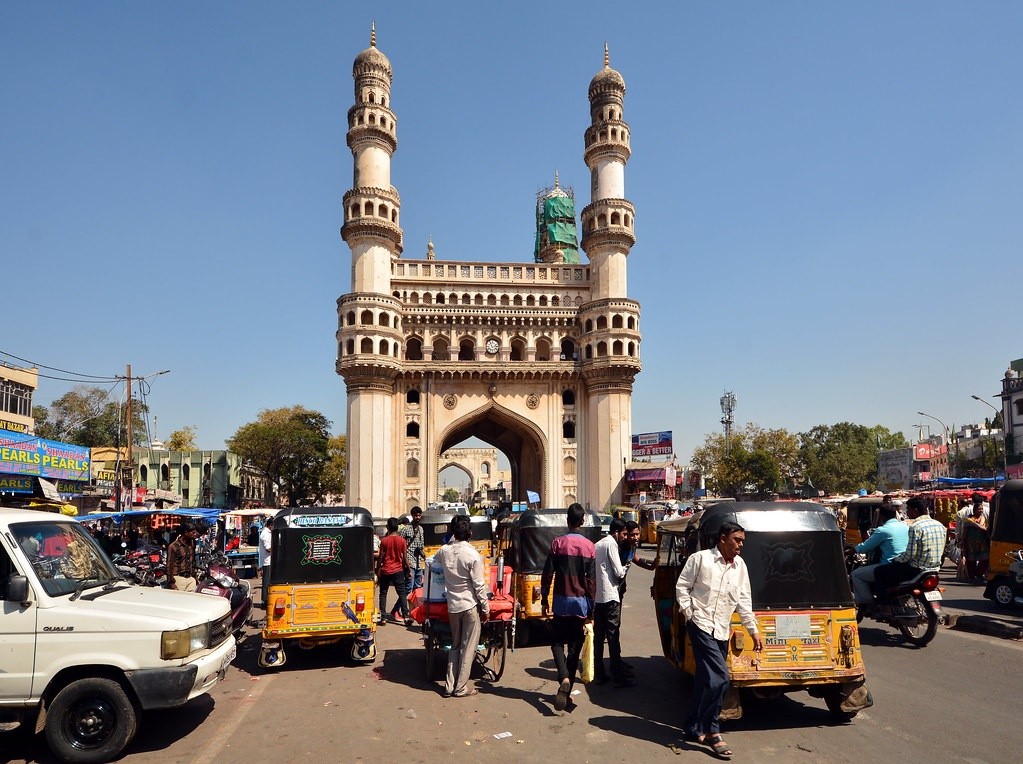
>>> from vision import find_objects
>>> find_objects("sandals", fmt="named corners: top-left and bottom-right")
top-left (704, 736), bottom-right (732, 757)
top-left (458, 689), bottom-right (478, 696)
top-left (444, 692), bottom-right (453, 697)
top-left (682, 729), bottom-right (707, 745)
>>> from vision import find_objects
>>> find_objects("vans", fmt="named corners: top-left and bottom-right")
top-left (429, 502), bottom-right (471, 516)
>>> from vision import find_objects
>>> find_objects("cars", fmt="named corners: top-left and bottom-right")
top-left (0, 507), bottom-right (237, 764)
top-left (596, 513), bottom-right (615, 536)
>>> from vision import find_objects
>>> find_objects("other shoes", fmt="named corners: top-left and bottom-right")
top-left (390, 610), bottom-right (403, 621)
top-left (554, 683), bottom-right (570, 711)
top-left (856, 608), bottom-right (870, 624)
top-left (594, 674), bottom-right (611, 683)
top-left (612, 679), bottom-right (637, 688)
top-left (873, 607), bottom-right (892, 618)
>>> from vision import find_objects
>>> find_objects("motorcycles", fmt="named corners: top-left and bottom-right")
top-left (650, 501), bottom-right (874, 721)
top-left (983, 480), bottom-right (1023, 609)
top-left (399, 509), bottom-right (602, 682)
top-left (111, 541), bottom-right (167, 588)
top-left (843, 498), bottom-right (947, 647)
top-left (616, 498), bottom-right (736, 548)
top-left (258, 506), bottom-right (381, 667)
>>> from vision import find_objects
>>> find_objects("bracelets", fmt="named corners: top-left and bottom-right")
top-left (541, 599), bottom-right (548, 605)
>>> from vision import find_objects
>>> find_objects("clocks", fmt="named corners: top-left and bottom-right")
top-left (486, 339), bottom-right (499, 354)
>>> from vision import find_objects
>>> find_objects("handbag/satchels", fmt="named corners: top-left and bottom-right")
top-left (404, 564), bottom-right (410, 580)
top-left (582, 623), bottom-right (594, 683)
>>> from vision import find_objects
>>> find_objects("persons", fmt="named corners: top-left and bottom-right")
top-left (662, 502), bottom-right (704, 552)
top-left (434, 515), bottom-right (490, 697)
top-left (540, 503), bottom-right (596, 711)
top-left (373, 507), bottom-right (505, 626)
top-left (593, 518), bottom-right (662, 681)
top-left (837, 488), bottom-right (990, 624)
top-left (0, 490), bottom-right (273, 610)
top-left (675, 521), bottom-right (763, 757)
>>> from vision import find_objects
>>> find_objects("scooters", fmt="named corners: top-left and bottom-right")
top-left (192, 558), bottom-right (254, 640)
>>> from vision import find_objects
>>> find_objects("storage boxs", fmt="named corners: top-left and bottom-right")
top-left (240, 545), bottom-right (259, 552)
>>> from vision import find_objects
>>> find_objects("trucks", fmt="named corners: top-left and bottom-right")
top-left (224, 509), bottom-right (283, 579)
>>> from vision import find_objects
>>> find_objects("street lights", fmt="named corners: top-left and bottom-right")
top-left (912, 412), bottom-right (950, 479)
top-left (972, 394), bottom-right (1007, 471)
